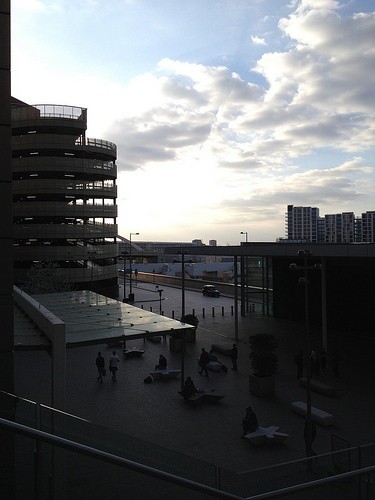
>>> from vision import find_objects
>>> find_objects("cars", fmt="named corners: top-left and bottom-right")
top-left (202, 285), bottom-right (220, 297)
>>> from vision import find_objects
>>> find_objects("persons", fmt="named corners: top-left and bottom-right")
top-left (109, 350), bottom-right (120, 384)
top-left (240, 406), bottom-right (258, 439)
top-left (95, 352), bottom-right (106, 386)
top-left (155, 354), bottom-right (168, 373)
top-left (231, 343), bottom-right (238, 372)
top-left (294, 346), bottom-right (330, 379)
top-left (177, 377), bottom-right (195, 398)
top-left (198, 347), bottom-right (218, 378)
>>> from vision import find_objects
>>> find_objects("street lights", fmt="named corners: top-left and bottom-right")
top-left (129, 232), bottom-right (139, 302)
top-left (240, 232), bottom-right (248, 242)
